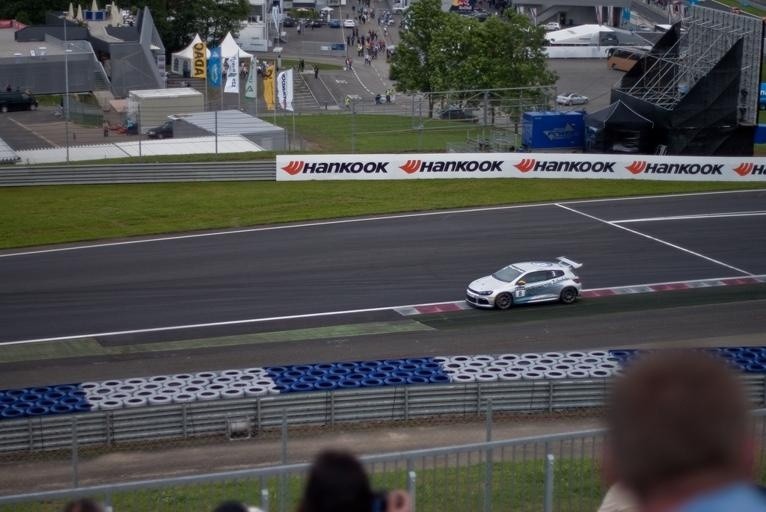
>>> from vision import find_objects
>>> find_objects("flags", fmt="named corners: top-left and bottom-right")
top-left (189, 41), bottom-right (295, 113)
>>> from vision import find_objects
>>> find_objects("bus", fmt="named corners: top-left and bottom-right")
top-left (605, 47), bottom-right (650, 72)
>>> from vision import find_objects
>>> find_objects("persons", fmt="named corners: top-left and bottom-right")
top-left (6, 84), bottom-right (32, 95)
top-left (103, 118), bottom-right (110, 137)
top-left (297, 56), bottom-right (305, 72)
top-left (343, 0), bottom-right (394, 71)
top-left (479, 0), bottom-right (505, 15)
top-left (310, 20), bottom-right (314, 31)
top-left (374, 88), bottom-right (393, 105)
top-left (314, 64), bottom-right (319, 79)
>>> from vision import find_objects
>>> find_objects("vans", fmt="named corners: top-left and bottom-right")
top-left (0, 91), bottom-right (38, 113)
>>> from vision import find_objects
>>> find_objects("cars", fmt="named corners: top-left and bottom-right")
top-left (465, 255), bottom-right (583, 310)
top-left (147, 121), bottom-right (173, 139)
top-left (636, 23), bottom-right (651, 32)
top-left (556, 91), bottom-right (589, 107)
top-left (437, 105), bottom-right (479, 122)
top-left (283, 18), bottom-right (356, 28)
top-left (539, 22), bottom-right (560, 31)
